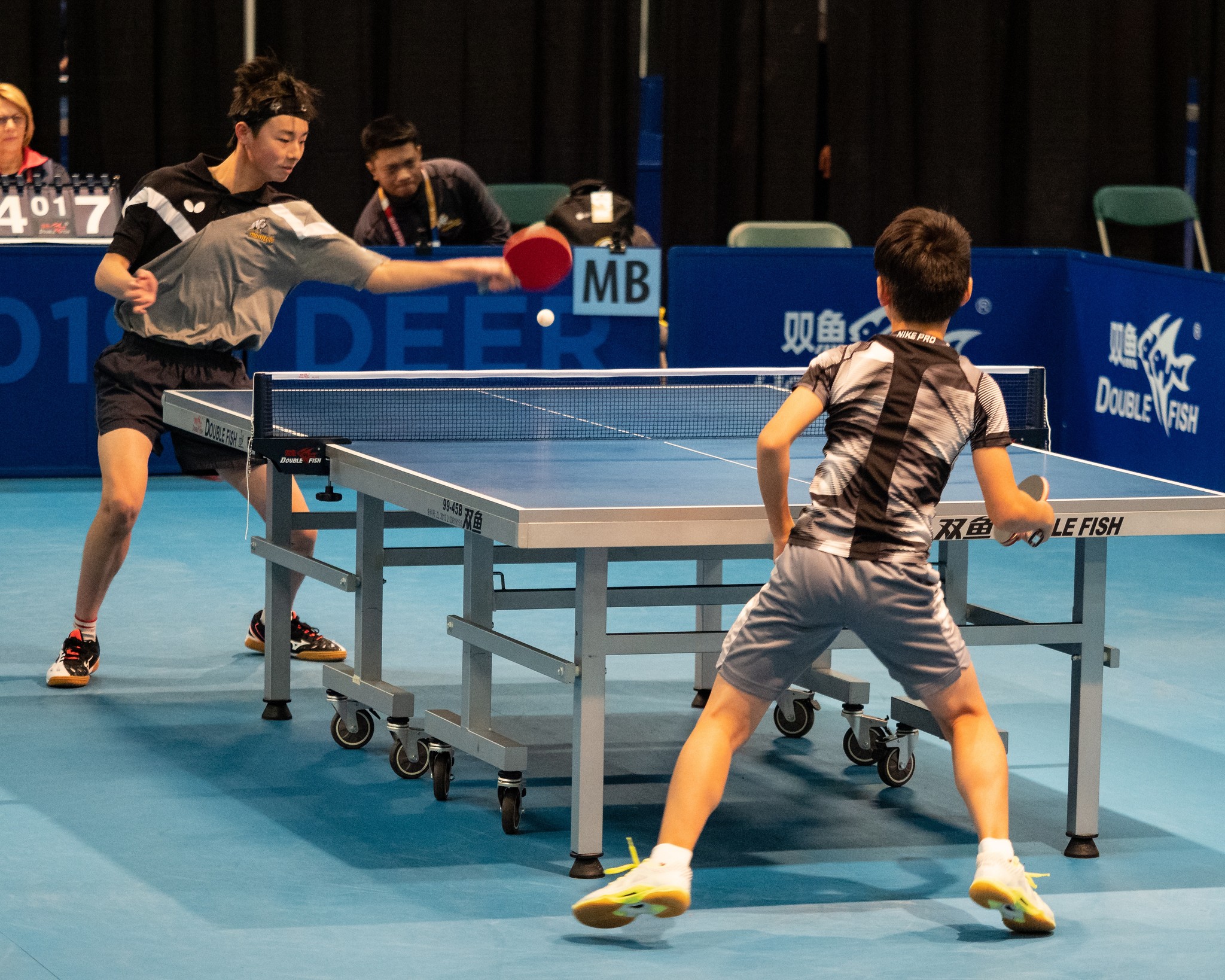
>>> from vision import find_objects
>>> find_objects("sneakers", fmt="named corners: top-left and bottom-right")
top-left (244, 609), bottom-right (348, 661)
top-left (46, 629), bottom-right (101, 686)
top-left (969, 852), bottom-right (1055, 931)
top-left (572, 837), bottom-right (691, 928)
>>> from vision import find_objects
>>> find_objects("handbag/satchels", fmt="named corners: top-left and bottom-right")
top-left (546, 180), bottom-right (634, 249)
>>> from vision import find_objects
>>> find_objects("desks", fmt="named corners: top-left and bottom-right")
top-left (161, 386), bottom-right (1225, 879)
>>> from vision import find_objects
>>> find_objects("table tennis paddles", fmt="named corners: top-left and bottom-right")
top-left (994, 475), bottom-right (1050, 548)
top-left (478, 226), bottom-right (573, 292)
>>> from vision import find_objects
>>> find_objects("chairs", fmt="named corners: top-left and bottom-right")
top-left (1094, 188), bottom-right (1211, 276)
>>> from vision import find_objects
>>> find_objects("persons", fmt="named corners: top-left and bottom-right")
top-left (47, 57), bottom-right (522, 685)
top-left (570, 181), bottom-right (655, 248)
top-left (0, 82), bottom-right (71, 185)
top-left (571, 204), bottom-right (1059, 936)
top-left (819, 144), bottom-right (832, 187)
top-left (354, 115), bottom-right (514, 245)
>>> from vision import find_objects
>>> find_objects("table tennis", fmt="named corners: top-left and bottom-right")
top-left (536, 309), bottom-right (554, 328)
